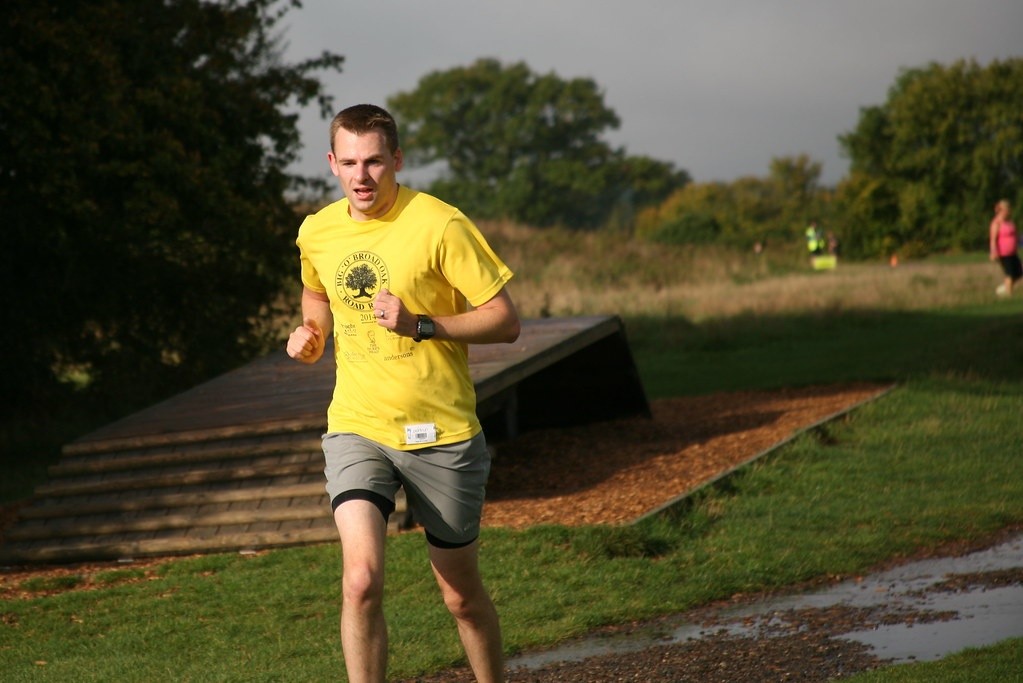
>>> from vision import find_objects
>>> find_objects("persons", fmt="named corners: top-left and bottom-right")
top-left (805, 221), bottom-right (840, 263)
top-left (287, 105), bottom-right (520, 683)
top-left (990, 200), bottom-right (1023, 294)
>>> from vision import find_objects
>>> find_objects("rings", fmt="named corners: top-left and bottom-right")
top-left (381, 311), bottom-right (384, 317)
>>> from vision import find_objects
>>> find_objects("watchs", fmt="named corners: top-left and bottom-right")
top-left (414, 314), bottom-right (435, 342)
